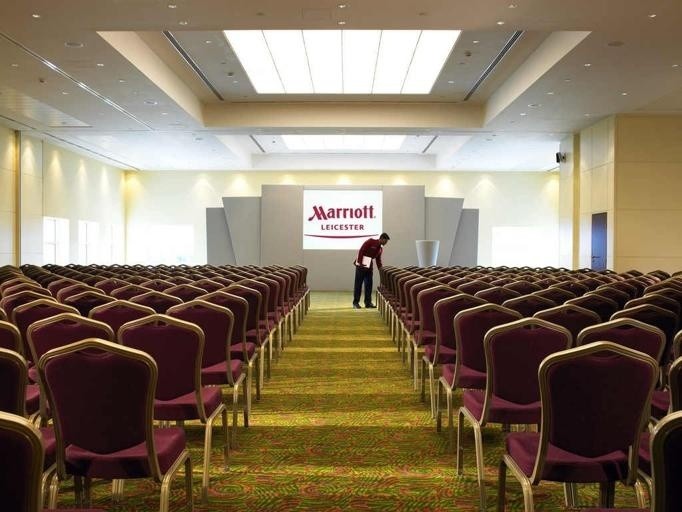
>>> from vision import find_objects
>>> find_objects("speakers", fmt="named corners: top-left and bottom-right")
top-left (556, 152), bottom-right (561, 163)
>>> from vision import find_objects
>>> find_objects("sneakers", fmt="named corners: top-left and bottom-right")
top-left (365, 304), bottom-right (377, 309)
top-left (353, 302), bottom-right (361, 309)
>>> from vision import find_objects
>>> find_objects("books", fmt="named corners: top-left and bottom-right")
top-left (355, 256), bottom-right (372, 268)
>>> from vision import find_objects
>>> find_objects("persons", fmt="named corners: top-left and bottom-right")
top-left (353, 233), bottom-right (390, 309)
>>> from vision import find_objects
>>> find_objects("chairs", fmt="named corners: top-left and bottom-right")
top-left (1, 286), bottom-right (263, 450)
top-left (1, 314), bottom-right (230, 511)
top-left (412, 285), bottom-right (682, 453)
top-left (456, 318), bottom-right (682, 512)
top-left (0, 263), bottom-right (310, 379)
top-left (376, 266), bottom-right (682, 372)
